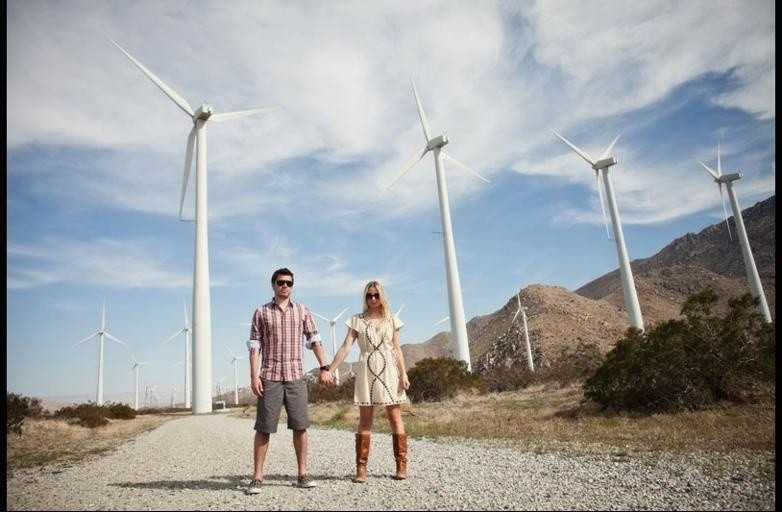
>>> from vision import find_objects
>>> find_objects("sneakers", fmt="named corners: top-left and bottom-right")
top-left (297, 473), bottom-right (316, 488)
top-left (246, 479), bottom-right (262, 495)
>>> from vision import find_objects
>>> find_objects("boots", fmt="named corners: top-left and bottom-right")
top-left (352, 432), bottom-right (371, 484)
top-left (391, 432), bottom-right (408, 479)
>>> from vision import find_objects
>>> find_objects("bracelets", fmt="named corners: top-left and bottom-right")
top-left (319, 365), bottom-right (330, 372)
top-left (400, 372), bottom-right (408, 380)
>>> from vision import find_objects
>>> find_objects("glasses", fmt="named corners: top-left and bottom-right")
top-left (275, 280), bottom-right (293, 288)
top-left (366, 292), bottom-right (380, 301)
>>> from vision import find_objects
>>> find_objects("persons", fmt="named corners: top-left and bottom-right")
top-left (318, 280), bottom-right (414, 484)
top-left (243, 268), bottom-right (334, 494)
